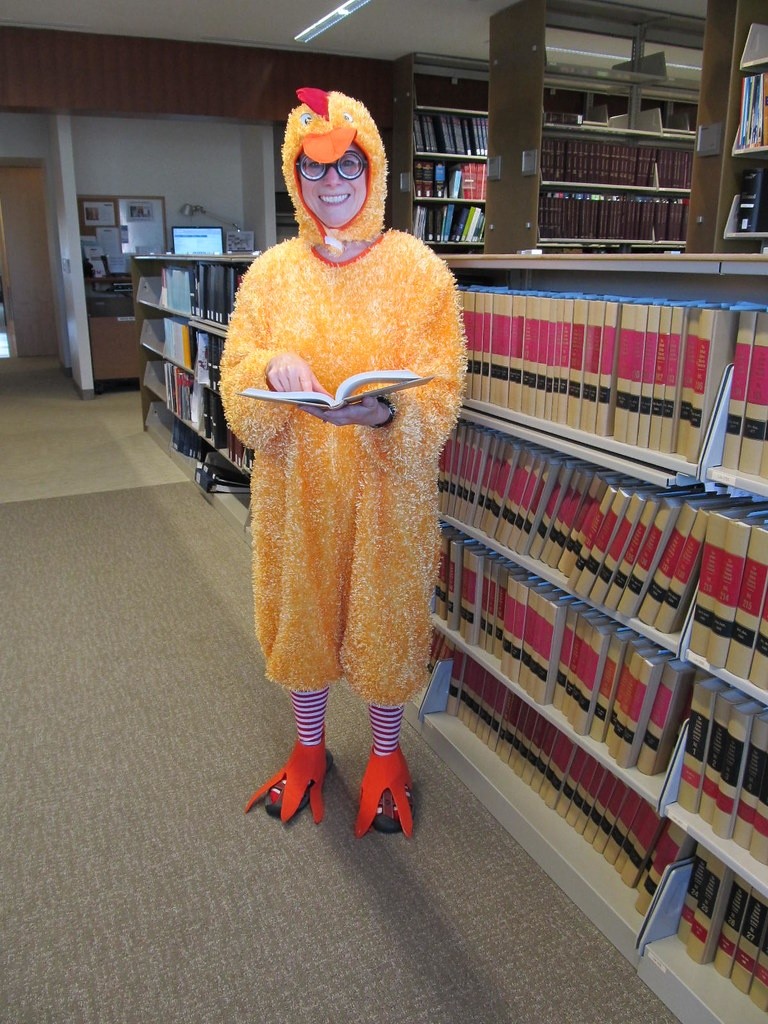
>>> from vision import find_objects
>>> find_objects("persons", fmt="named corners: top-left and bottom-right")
top-left (218, 87), bottom-right (469, 838)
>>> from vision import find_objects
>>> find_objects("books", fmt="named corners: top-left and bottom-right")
top-left (161, 261), bottom-right (255, 494)
top-left (238, 366), bottom-right (435, 411)
top-left (423, 285), bottom-right (768, 1012)
top-left (538, 138), bottom-right (694, 241)
top-left (737, 72), bottom-right (768, 232)
top-left (413, 111), bottom-right (488, 243)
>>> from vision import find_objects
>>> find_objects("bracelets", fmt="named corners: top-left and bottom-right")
top-left (369, 396), bottom-right (395, 428)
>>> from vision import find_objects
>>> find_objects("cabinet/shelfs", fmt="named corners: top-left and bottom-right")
top-left (399, 253), bottom-right (768, 1024)
top-left (483, 0), bottom-right (707, 254)
top-left (392, 52), bottom-right (702, 256)
top-left (129, 254), bottom-right (260, 551)
top-left (685, 0), bottom-right (768, 255)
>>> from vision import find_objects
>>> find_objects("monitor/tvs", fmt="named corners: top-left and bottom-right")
top-left (171, 226), bottom-right (225, 255)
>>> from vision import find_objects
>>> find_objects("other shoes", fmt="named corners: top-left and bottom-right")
top-left (371, 784), bottom-right (415, 833)
top-left (264, 748), bottom-right (333, 817)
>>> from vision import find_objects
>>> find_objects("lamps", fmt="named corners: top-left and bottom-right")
top-left (180, 203), bottom-right (242, 232)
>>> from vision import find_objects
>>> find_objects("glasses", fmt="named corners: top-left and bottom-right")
top-left (295, 150), bottom-right (368, 181)
